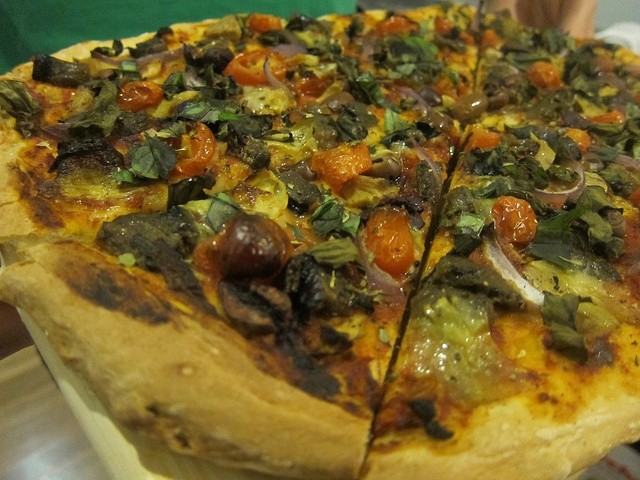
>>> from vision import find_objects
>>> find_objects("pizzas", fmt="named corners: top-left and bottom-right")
top-left (0, 7), bottom-right (640, 480)
